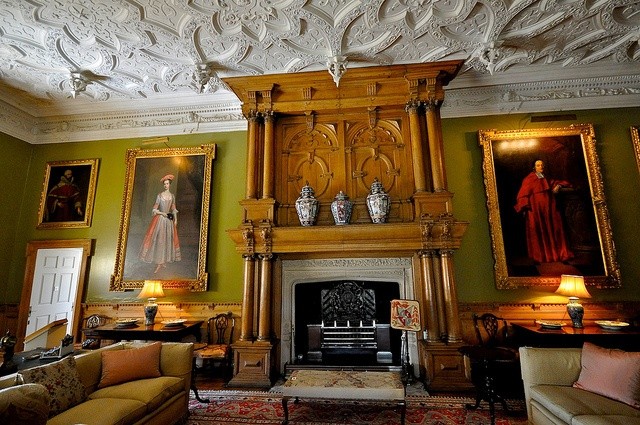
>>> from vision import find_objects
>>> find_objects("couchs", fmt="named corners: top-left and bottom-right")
top-left (518, 345), bottom-right (639, 424)
top-left (0, 342), bottom-right (194, 425)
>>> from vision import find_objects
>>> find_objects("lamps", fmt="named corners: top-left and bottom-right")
top-left (136, 280), bottom-right (165, 326)
top-left (390, 300), bottom-right (421, 385)
top-left (554, 275), bottom-right (592, 329)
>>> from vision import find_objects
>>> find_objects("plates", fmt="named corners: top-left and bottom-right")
top-left (161, 319), bottom-right (187, 327)
top-left (535, 320), bottom-right (566, 331)
top-left (115, 319), bottom-right (139, 327)
top-left (594, 320), bottom-right (629, 331)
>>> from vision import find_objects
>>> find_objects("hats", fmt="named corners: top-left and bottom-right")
top-left (160, 175), bottom-right (174, 182)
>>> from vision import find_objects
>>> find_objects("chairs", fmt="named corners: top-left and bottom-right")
top-left (471, 314), bottom-right (515, 424)
top-left (193, 312), bottom-right (236, 383)
top-left (80, 314), bottom-right (112, 347)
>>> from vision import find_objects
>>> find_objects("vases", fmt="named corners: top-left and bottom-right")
top-left (331, 189), bottom-right (353, 226)
top-left (367, 177), bottom-right (391, 224)
top-left (295, 181), bottom-right (319, 226)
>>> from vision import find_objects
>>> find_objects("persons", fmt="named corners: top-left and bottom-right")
top-left (138, 174), bottom-right (182, 274)
top-left (514, 159), bottom-right (576, 263)
top-left (44, 170), bottom-right (83, 222)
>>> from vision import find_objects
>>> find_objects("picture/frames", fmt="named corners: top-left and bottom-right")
top-left (35, 158), bottom-right (100, 229)
top-left (109, 141), bottom-right (216, 293)
top-left (478, 121), bottom-right (622, 288)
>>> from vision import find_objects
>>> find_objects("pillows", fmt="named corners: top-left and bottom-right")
top-left (97, 344), bottom-right (163, 389)
top-left (0, 384), bottom-right (47, 425)
top-left (19, 354), bottom-right (90, 417)
top-left (573, 339), bottom-right (639, 410)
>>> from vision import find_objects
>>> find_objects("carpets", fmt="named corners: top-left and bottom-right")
top-left (189, 389), bottom-right (526, 425)
